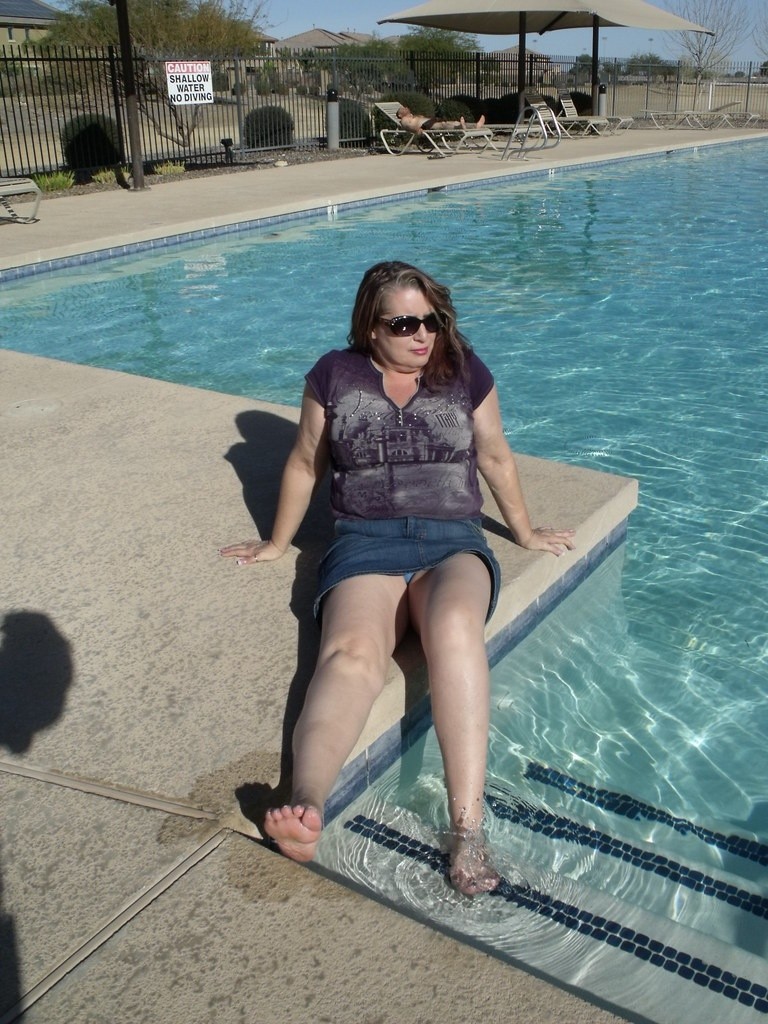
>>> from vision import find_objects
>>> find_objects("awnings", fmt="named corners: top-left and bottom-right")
top-left (375, 1), bottom-right (715, 135)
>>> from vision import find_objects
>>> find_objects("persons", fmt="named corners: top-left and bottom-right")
top-left (396, 104), bottom-right (486, 134)
top-left (216, 258), bottom-right (578, 865)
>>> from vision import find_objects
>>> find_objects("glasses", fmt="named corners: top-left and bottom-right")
top-left (376, 313), bottom-right (439, 337)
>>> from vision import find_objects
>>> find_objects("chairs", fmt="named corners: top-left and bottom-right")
top-left (523, 86), bottom-right (608, 138)
top-left (640, 101), bottom-right (761, 129)
top-left (443, 120), bottom-right (544, 150)
top-left (555, 82), bottom-right (634, 135)
top-left (374, 102), bottom-right (494, 158)
top-left (0, 178), bottom-right (42, 223)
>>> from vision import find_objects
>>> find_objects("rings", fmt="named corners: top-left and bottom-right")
top-left (254, 554), bottom-right (259, 564)
